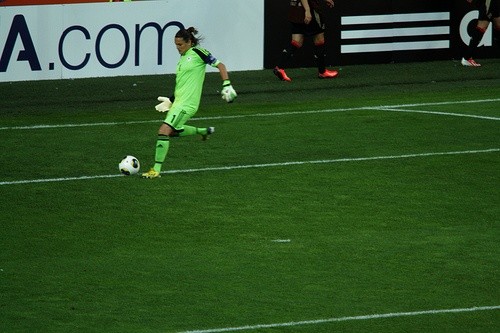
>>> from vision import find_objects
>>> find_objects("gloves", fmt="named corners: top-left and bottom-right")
top-left (155, 96), bottom-right (175, 113)
top-left (217, 79), bottom-right (238, 102)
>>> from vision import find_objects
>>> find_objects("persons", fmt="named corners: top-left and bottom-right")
top-left (461, 0), bottom-right (500, 66)
top-left (274, 0), bottom-right (339, 80)
top-left (141, 26), bottom-right (237, 178)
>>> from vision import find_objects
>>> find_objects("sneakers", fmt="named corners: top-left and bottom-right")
top-left (273, 66), bottom-right (292, 81)
top-left (142, 168), bottom-right (161, 179)
top-left (318, 69), bottom-right (339, 78)
top-left (202, 127), bottom-right (214, 141)
top-left (460, 57), bottom-right (481, 67)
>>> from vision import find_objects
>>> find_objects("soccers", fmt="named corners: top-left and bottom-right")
top-left (118, 156), bottom-right (140, 176)
